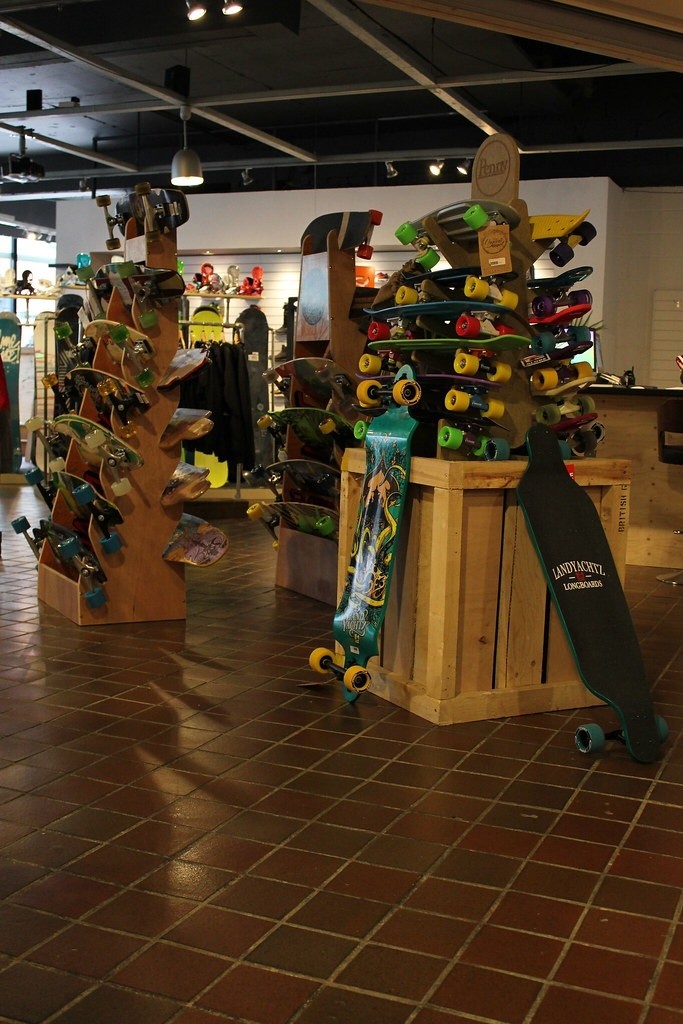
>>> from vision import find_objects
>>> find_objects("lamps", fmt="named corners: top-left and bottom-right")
top-left (457, 158), bottom-right (471, 175)
top-left (429, 159), bottom-right (447, 175)
top-left (171, 104), bottom-right (205, 186)
top-left (385, 161), bottom-right (398, 178)
top-left (222, 0), bottom-right (243, 15)
top-left (241, 168), bottom-right (254, 186)
top-left (186, 0), bottom-right (207, 21)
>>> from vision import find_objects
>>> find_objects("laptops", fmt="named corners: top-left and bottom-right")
top-left (75, 251), bottom-right (124, 286)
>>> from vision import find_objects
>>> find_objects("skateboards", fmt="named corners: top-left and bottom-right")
top-left (8, 183), bottom-right (607, 612)
top-left (307, 362), bottom-right (423, 706)
top-left (512, 421), bottom-right (672, 763)
